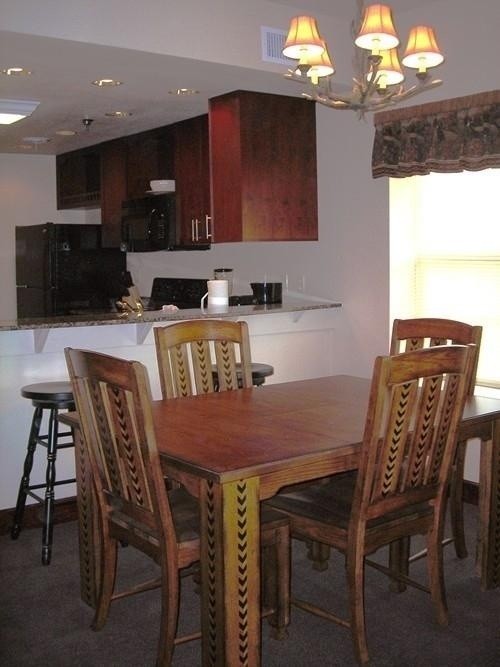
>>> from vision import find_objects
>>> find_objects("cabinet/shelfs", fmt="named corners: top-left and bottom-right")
top-left (0, 292), bottom-right (344, 517)
top-left (11, 223), bottom-right (128, 326)
top-left (56, 89), bottom-right (317, 249)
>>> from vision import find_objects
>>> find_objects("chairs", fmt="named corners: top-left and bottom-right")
top-left (65, 348), bottom-right (291, 667)
top-left (152, 320), bottom-right (253, 401)
top-left (387, 319), bottom-right (480, 398)
top-left (254, 343), bottom-right (476, 667)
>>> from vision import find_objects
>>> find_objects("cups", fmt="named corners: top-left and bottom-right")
top-left (266, 282), bottom-right (283, 305)
top-left (249, 282), bottom-right (265, 305)
top-left (201, 279), bottom-right (230, 314)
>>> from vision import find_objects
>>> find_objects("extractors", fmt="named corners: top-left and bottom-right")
top-left (120, 197), bottom-right (170, 252)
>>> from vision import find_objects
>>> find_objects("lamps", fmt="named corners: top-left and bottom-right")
top-left (170, 87), bottom-right (196, 97)
top-left (56, 130), bottom-right (76, 136)
top-left (23, 136), bottom-right (45, 141)
top-left (284, 0), bottom-right (445, 120)
top-left (93, 77), bottom-right (120, 90)
top-left (0, 97), bottom-right (36, 125)
top-left (6, 65), bottom-right (27, 76)
top-left (107, 112), bottom-right (129, 118)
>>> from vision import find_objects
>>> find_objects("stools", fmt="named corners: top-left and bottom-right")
top-left (10, 381), bottom-right (136, 566)
top-left (204, 363), bottom-right (273, 391)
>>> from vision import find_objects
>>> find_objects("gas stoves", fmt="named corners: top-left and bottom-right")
top-left (141, 277), bottom-right (207, 312)
